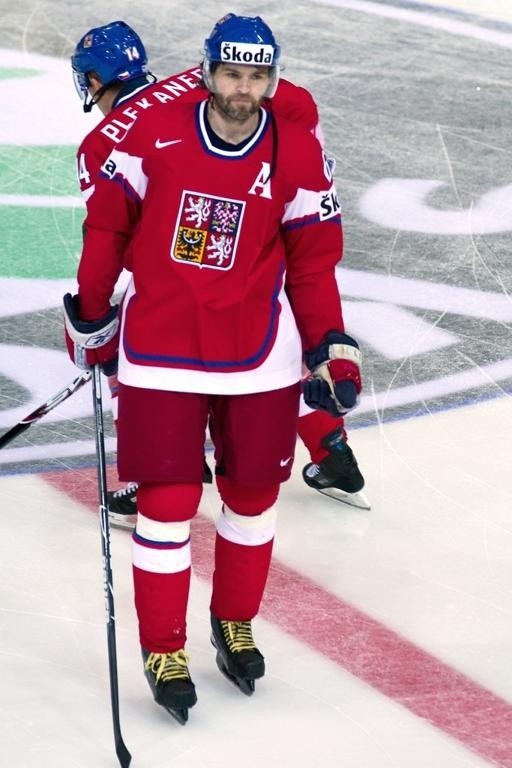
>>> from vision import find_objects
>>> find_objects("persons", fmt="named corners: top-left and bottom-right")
top-left (63, 12), bottom-right (363, 723)
top-left (69, 19), bottom-right (371, 528)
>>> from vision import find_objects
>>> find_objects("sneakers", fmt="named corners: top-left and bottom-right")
top-left (105, 483), bottom-right (138, 516)
top-left (140, 650), bottom-right (197, 703)
top-left (320, 446), bottom-right (365, 494)
top-left (210, 613), bottom-right (265, 681)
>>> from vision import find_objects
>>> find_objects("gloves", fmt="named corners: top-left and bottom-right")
top-left (62, 292), bottom-right (124, 371)
top-left (304, 327), bottom-right (363, 418)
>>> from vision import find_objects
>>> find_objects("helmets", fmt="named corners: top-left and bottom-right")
top-left (203, 11), bottom-right (281, 100)
top-left (72, 20), bottom-right (148, 99)
